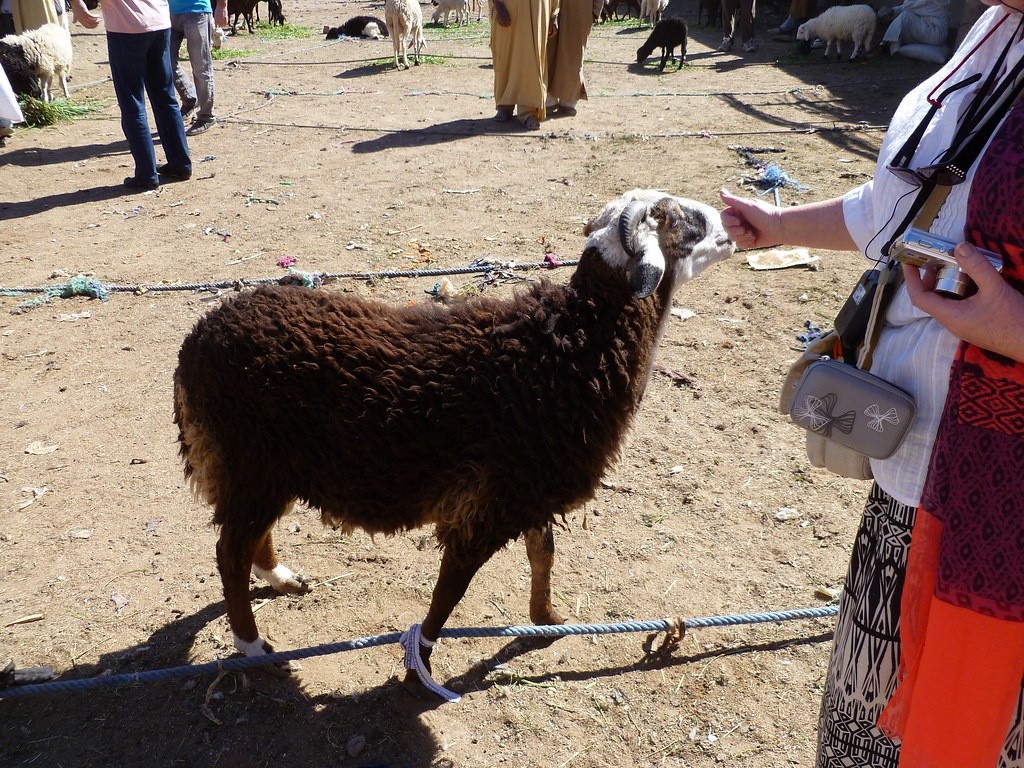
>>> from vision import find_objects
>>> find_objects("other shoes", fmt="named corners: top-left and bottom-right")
top-left (155, 162), bottom-right (193, 177)
top-left (525, 116), bottom-right (542, 130)
top-left (766, 26), bottom-right (784, 35)
top-left (124, 177), bottom-right (160, 189)
top-left (547, 102), bottom-right (559, 111)
top-left (559, 104), bottom-right (576, 116)
top-left (494, 105), bottom-right (514, 121)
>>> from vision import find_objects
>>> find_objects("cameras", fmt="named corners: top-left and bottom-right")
top-left (889, 228), bottom-right (1002, 300)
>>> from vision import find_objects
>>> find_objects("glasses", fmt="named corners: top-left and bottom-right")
top-left (885, 73), bottom-right (1024, 186)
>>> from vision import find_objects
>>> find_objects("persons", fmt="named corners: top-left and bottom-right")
top-left (720, 0), bottom-right (1024, 768)
top-left (488, 0), bottom-right (605, 129)
top-left (168, 0), bottom-right (228, 136)
top-left (11, 0), bottom-right (66, 83)
top-left (876, 0), bottom-right (952, 59)
top-left (766, 0), bottom-right (815, 42)
top-left (717, 0), bottom-right (756, 53)
top-left (70, 0), bottom-right (192, 189)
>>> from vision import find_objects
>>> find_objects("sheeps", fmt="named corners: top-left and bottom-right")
top-left (796, 4), bottom-right (878, 61)
top-left (385, 0), bottom-right (427, 70)
top-left (326, 15), bottom-right (388, 43)
top-left (209, 0), bottom-right (287, 34)
top-left (595, 0), bottom-right (689, 76)
top-left (430, 0), bottom-right (485, 28)
top-left (0, 22), bottom-right (74, 105)
top-left (171, 188), bottom-right (738, 671)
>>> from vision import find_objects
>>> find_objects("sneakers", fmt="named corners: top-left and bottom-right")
top-left (188, 118), bottom-right (217, 136)
top-left (181, 98), bottom-right (200, 120)
top-left (718, 37), bottom-right (734, 51)
top-left (743, 41), bottom-right (755, 52)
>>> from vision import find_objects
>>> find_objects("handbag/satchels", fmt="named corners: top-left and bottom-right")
top-left (780, 329), bottom-right (919, 479)
